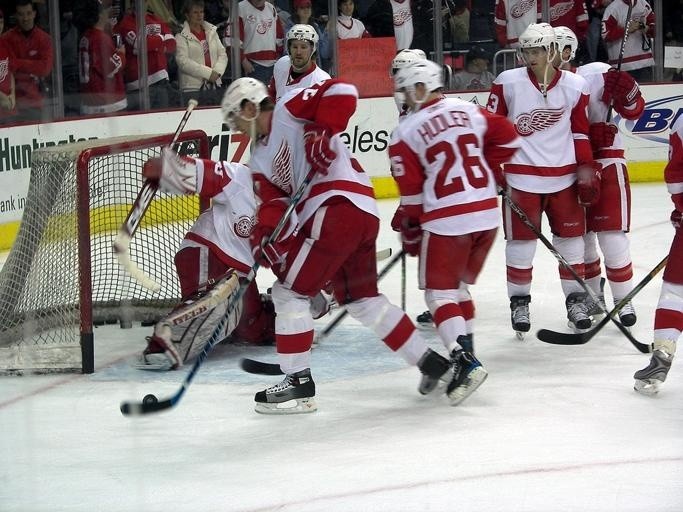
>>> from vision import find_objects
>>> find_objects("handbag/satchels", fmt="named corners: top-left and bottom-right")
top-left (182, 87), bottom-right (223, 106)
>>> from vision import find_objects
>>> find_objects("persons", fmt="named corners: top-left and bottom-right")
top-left (369, 2), bottom-right (422, 55)
top-left (447, 2), bottom-right (471, 43)
top-left (485, 23), bottom-right (645, 342)
top-left (173, 2), bottom-right (228, 107)
top-left (634, 113), bottom-right (683, 398)
top-left (495, 2), bottom-right (543, 58)
top-left (537, 1), bottom-right (590, 67)
top-left (282, 1), bottom-right (330, 68)
top-left (390, 48), bottom-right (524, 405)
top-left (336, 2), bottom-right (372, 40)
top-left (451, 46), bottom-right (497, 90)
top-left (133, 23), bottom-right (455, 415)
top-left (601, 1), bottom-right (656, 83)
top-left (588, 2), bottom-right (608, 62)
top-left (117, 1), bottom-right (177, 110)
top-left (1, 1), bottom-right (56, 125)
top-left (223, 1), bottom-right (285, 88)
top-left (77, 1), bottom-right (129, 116)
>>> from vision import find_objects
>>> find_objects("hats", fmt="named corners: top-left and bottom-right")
top-left (470, 44), bottom-right (494, 60)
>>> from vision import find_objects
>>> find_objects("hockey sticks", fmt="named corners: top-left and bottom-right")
top-left (120, 163), bottom-right (317, 416)
top-left (496, 186), bottom-right (655, 354)
top-left (240, 251), bottom-right (405, 375)
top-left (112, 99), bottom-right (197, 291)
top-left (537, 256), bottom-right (670, 345)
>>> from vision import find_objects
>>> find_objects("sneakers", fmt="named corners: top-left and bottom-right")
top-left (635, 349), bottom-right (672, 382)
top-left (510, 294), bottom-right (638, 332)
top-left (256, 368), bottom-right (315, 403)
top-left (416, 310), bottom-right (483, 395)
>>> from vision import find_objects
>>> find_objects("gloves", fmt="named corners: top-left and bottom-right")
top-left (402, 227), bottom-right (422, 257)
top-left (576, 163), bottom-right (603, 207)
top-left (590, 122), bottom-right (617, 146)
top-left (604, 70), bottom-right (641, 106)
top-left (248, 223), bottom-right (289, 269)
top-left (304, 123), bottom-right (336, 176)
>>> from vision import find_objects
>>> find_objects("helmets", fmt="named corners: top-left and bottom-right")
top-left (391, 49), bottom-right (444, 105)
top-left (284, 24), bottom-right (319, 57)
top-left (519, 23), bottom-right (578, 61)
top-left (222, 77), bottom-right (269, 125)
